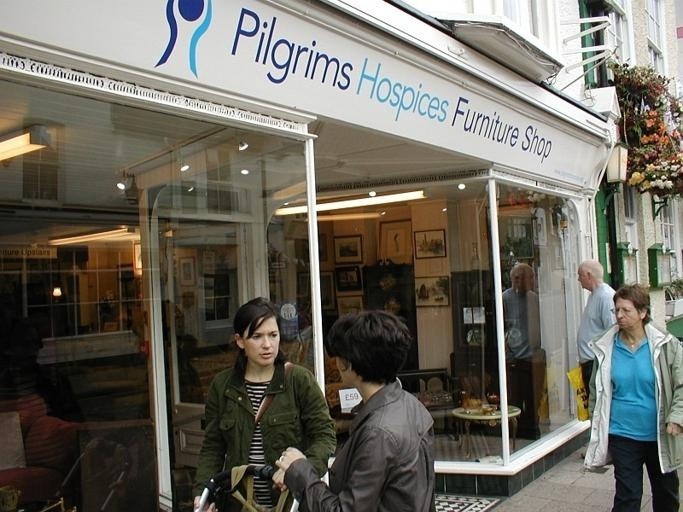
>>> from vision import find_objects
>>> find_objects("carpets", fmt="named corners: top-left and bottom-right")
top-left (434, 490), bottom-right (504, 511)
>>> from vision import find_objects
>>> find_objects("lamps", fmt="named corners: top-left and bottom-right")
top-left (272, 188), bottom-right (428, 216)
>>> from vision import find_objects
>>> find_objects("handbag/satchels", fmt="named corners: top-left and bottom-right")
top-left (566, 366), bottom-right (589, 421)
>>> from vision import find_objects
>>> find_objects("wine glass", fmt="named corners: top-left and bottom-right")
top-left (459, 390), bottom-right (472, 414)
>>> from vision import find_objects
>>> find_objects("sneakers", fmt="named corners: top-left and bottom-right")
top-left (581, 450), bottom-right (613, 465)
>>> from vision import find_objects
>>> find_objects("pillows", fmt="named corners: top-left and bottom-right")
top-left (1, 409), bottom-right (29, 472)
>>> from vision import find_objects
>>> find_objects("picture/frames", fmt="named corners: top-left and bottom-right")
top-left (134, 243), bottom-right (143, 269)
top-left (178, 255), bottom-right (196, 287)
top-left (333, 219), bottom-right (450, 307)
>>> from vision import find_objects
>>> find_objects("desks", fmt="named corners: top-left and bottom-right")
top-left (451, 406), bottom-right (523, 459)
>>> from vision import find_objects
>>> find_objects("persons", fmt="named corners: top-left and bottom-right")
top-left (589, 283), bottom-right (683, 512)
top-left (577, 260), bottom-right (616, 395)
top-left (275, 310), bottom-right (435, 512)
top-left (192, 297), bottom-right (336, 512)
top-left (502, 263), bottom-right (547, 439)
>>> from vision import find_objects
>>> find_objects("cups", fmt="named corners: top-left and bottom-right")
top-left (481, 404), bottom-right (497, 416)
top-left (486, 391), bottom-right (501, 411)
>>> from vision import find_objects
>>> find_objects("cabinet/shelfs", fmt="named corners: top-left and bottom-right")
top-left (449, 269), bottom-right (541, 440)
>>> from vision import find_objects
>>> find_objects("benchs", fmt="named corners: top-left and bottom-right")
top-left (396, 369), bottom-right (464, 436)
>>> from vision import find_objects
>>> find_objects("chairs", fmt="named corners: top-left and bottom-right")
top-left (0, 394), bottom-right (84, 511)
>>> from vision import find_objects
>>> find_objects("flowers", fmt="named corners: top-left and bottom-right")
top-left (605, 54), bottom-right (682, 204)
